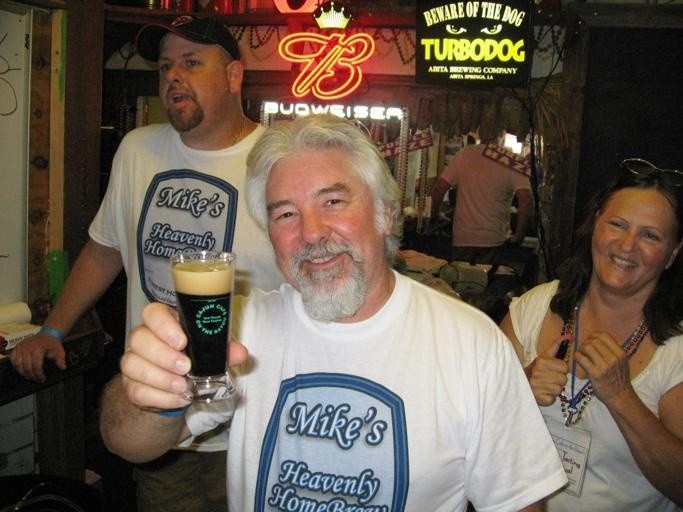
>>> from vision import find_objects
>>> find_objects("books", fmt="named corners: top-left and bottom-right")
top-left (0, 321), bottom-right (42, 352)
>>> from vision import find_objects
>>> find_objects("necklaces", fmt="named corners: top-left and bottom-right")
top-left (118, 104), bottom-right (130, 141)
top-left (396, 110), bottom-right (409, 217)
top-left (554, 300), bottom-right (654, 427)
top-left (415, 129), bottom-right (431, 233)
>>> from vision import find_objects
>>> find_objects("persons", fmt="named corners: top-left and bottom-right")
top-left (487, 159), bottom-right (682, 512)
top-left (95, 112), bottom-right (569, 511)
top-left (6, 10), bottom-right (268, 511)
top-left (428, 116), bottom-right (529, 266)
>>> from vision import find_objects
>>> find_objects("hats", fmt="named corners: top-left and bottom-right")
top-left (135, 13), bottom-right (241, 61)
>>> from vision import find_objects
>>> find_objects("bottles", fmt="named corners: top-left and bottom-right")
top-left (46, 250), bottom-right (70, 305)
top-left (439, 263), bottom-right (489, 295)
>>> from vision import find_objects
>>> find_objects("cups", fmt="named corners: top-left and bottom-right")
top-left (171, 249), bottom-right (236, 401)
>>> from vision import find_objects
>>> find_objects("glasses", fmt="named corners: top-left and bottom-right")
top-left (619, 158), bottom-right (683, 187)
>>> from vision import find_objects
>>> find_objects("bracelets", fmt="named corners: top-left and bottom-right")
top-left (38, 326), bottom-right (67, 342)
top-left (157, 404), bottom-right (188, 420)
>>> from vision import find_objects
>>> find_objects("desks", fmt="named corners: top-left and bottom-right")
top-left (0, 308), bottom-right (106, 406)
